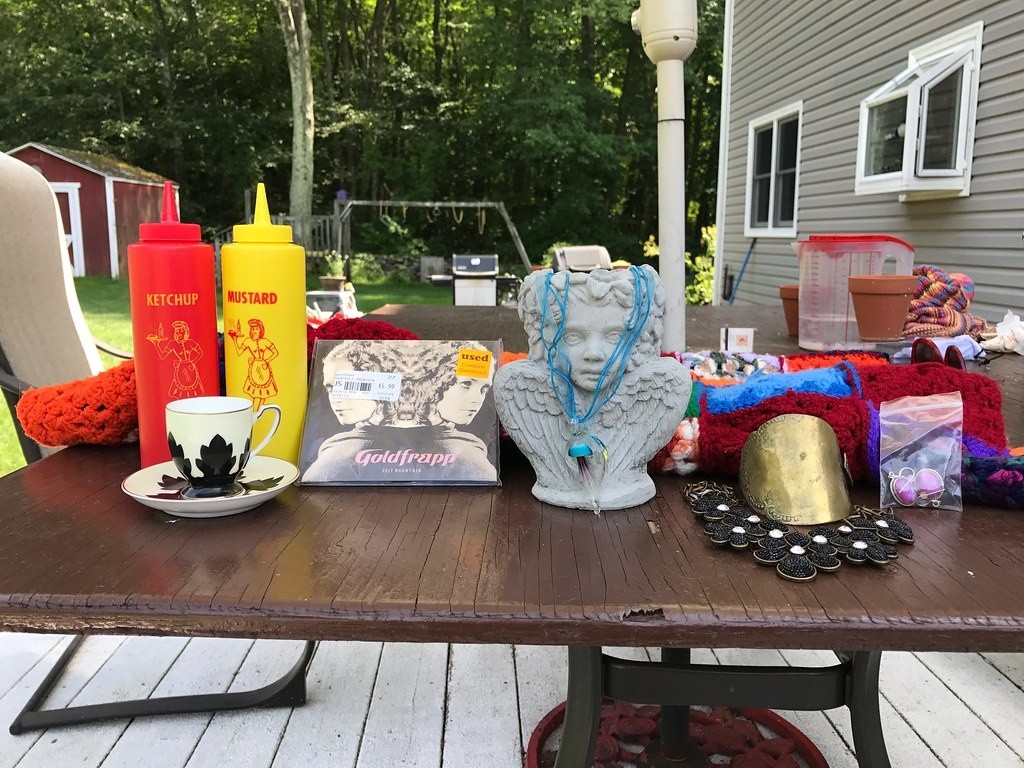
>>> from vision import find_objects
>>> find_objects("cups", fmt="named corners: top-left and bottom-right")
top-left (165, 395), bottom-right (282, 501)
top-left (849, 275), bottom-right (918, 341)
top-left (780, 285), bottom-right (801, 336)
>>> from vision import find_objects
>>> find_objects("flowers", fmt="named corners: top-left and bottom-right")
top-left (318, 250), bottom-right (349, 275)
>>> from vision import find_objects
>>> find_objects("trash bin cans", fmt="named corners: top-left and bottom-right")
top-left (450, 251), bottom-right (501, 307)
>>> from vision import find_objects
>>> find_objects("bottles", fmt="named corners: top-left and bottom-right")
top-left (220, 181), bottom-right (307, 467)
top-left (127, 180), bottom-right (219, 469)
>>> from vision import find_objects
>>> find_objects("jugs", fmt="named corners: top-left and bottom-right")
top-left (789, 234), bottom-right (915, 352)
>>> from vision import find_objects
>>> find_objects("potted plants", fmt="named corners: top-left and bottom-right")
top-left (530, 240), bottom-right (632, 273)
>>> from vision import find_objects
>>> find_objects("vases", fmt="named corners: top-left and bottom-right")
top-left (319, 276), bottom-right (346, 290)
top-left (849, 275), bottom-right (919, 342)
top-left (779, 284), bottom-right (828, 336)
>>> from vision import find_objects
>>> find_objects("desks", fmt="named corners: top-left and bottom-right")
top-left (0, 303), bottom-right (1024, 768)
top-left (306, 289), bottom-right (356, 312)
top-left (497, 277), bottom-right (521, 306)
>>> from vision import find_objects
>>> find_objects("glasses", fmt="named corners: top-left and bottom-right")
top-left (911, 338), bottom-right (968, 374)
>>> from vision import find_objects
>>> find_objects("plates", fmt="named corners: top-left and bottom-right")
top-left (121, 454), bottom-right (300, 518)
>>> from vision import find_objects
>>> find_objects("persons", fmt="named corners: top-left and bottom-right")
top-left (337, 281), bottom-right (367, 319)
top-left (493, 264), bottom-right (693, 510)
top-left (301, 340), bottom-right (498, 482)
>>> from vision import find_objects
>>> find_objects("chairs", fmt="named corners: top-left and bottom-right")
top-left (1, 152), bottom-right (315, 734)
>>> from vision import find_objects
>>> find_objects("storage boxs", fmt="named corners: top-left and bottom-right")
top-left (790, 234), bottom-right (914, 351)
top-left (720, 327), bottom-right (754, 353)
